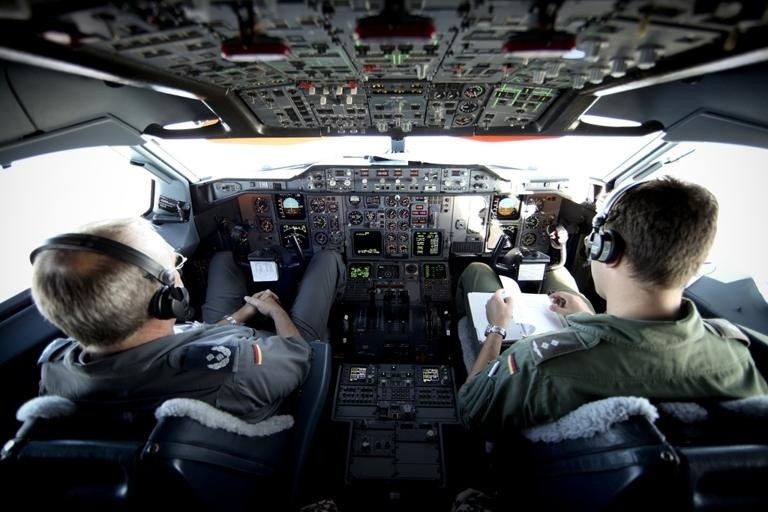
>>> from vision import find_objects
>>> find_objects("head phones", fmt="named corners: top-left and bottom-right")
top-left (585, 178), bottom-right (651, 265)
top-left (28, 230), bottom-right (195, 323)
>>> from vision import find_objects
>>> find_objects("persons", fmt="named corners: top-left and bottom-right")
top-left (452, 174), bottom-right (768, 433)
top-left (27, 215), bottom-right (348, 431)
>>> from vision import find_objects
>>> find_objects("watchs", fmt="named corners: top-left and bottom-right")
top-left (483, 324), bottom-right (507, 339)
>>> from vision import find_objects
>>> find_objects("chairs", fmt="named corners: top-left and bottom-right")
top-left (0, 339), bottom-right (335, 512)
top-left (449, 316), bottom-right (768, 512)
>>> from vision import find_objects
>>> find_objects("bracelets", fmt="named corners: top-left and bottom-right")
top-left (223, 314), bottom-right (238, 326)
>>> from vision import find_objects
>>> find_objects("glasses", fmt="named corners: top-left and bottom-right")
top-left (171, 251), bottom-right (188, 273)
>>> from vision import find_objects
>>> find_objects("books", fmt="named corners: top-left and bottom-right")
top-left (466, 289), bottom-right (568, 348)
